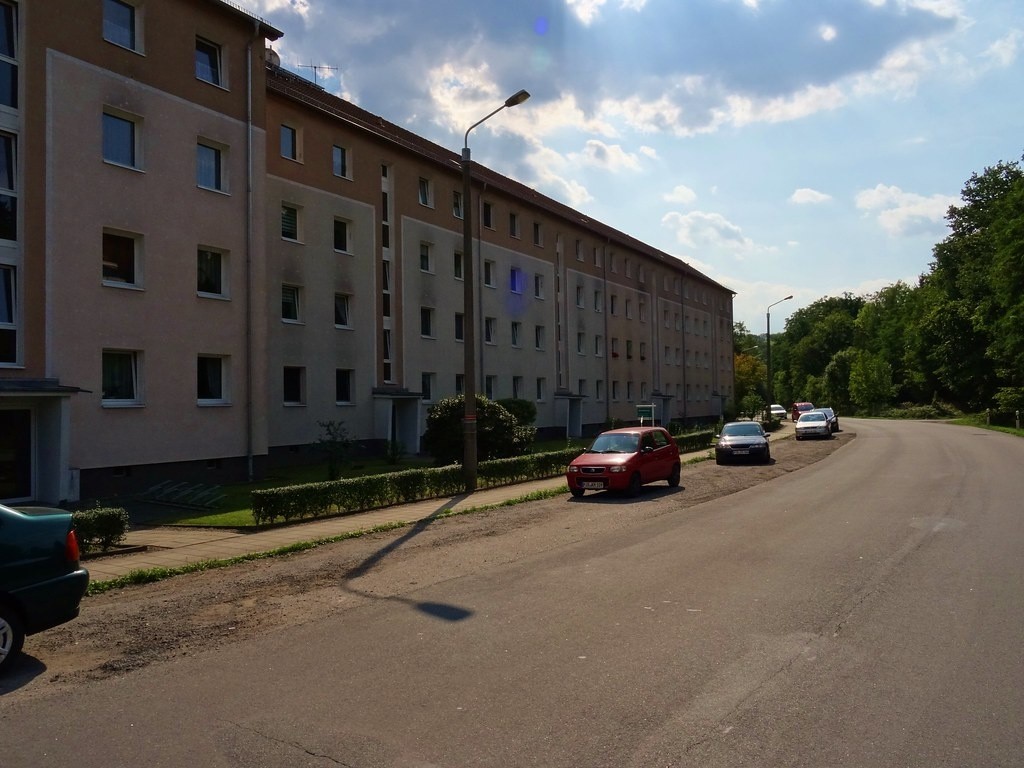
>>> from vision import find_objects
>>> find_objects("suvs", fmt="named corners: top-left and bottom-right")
top-left (810, 407), bottom-right (839, 431)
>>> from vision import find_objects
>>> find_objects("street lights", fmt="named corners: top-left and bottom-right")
top-left (767, 295), bottom-right (793, 404)
top-left (460, 89), bottom-right (532, 490)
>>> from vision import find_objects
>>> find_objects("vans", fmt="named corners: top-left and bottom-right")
top-left (792, 402), bottom-right (813, 421)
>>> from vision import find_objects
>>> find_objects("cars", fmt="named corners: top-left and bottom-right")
top-left (0, 503), bottom-right (90, 676)
top-left (794, 412), bottom-right (831, 440)
top-left (566, 426), bottom-right (681, 498)
top-left (715, 421), bottom-right (770, 464)
top-left (763, 404), bottom-right (787, 419)
